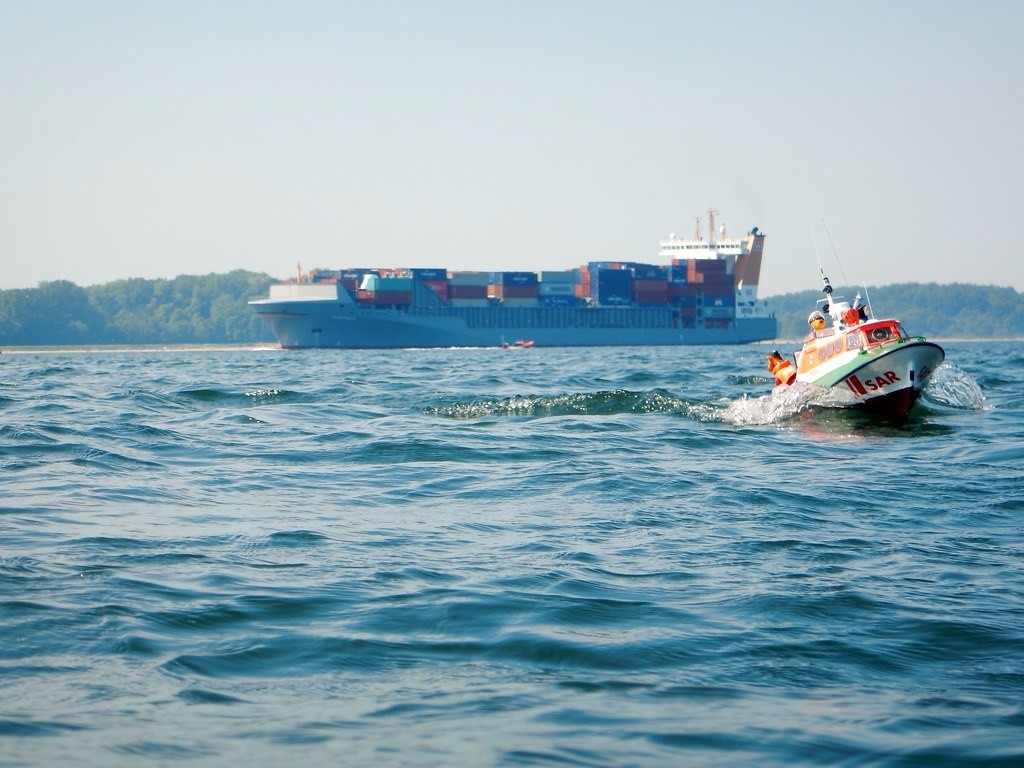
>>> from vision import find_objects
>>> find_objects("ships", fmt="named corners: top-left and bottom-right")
top-left (247, 206), bottom-right (778, 352)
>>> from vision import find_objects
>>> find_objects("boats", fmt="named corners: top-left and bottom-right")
top-left (499, 340), bottom-right (534, 350)
top-left (766, 264), bottom-right (946, 424)
